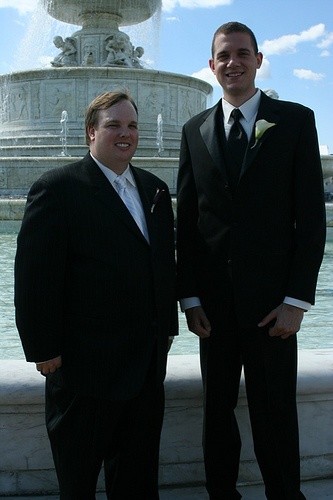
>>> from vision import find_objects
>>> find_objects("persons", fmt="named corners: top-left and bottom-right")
top-left (50, 33), bottom-right (145, 70)
top-left (175, 23), bottom-right (327, 499)
top-left (14, 90), bottom-right (177, 499)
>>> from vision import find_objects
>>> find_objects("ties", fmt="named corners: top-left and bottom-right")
top-left (226, 109), bottom-right (248, 189)
top-left (114, 177), bottom-right (149, 243)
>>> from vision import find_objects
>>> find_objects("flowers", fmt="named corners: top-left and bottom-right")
top-left (248, 118), bottom-right (276, 150)
